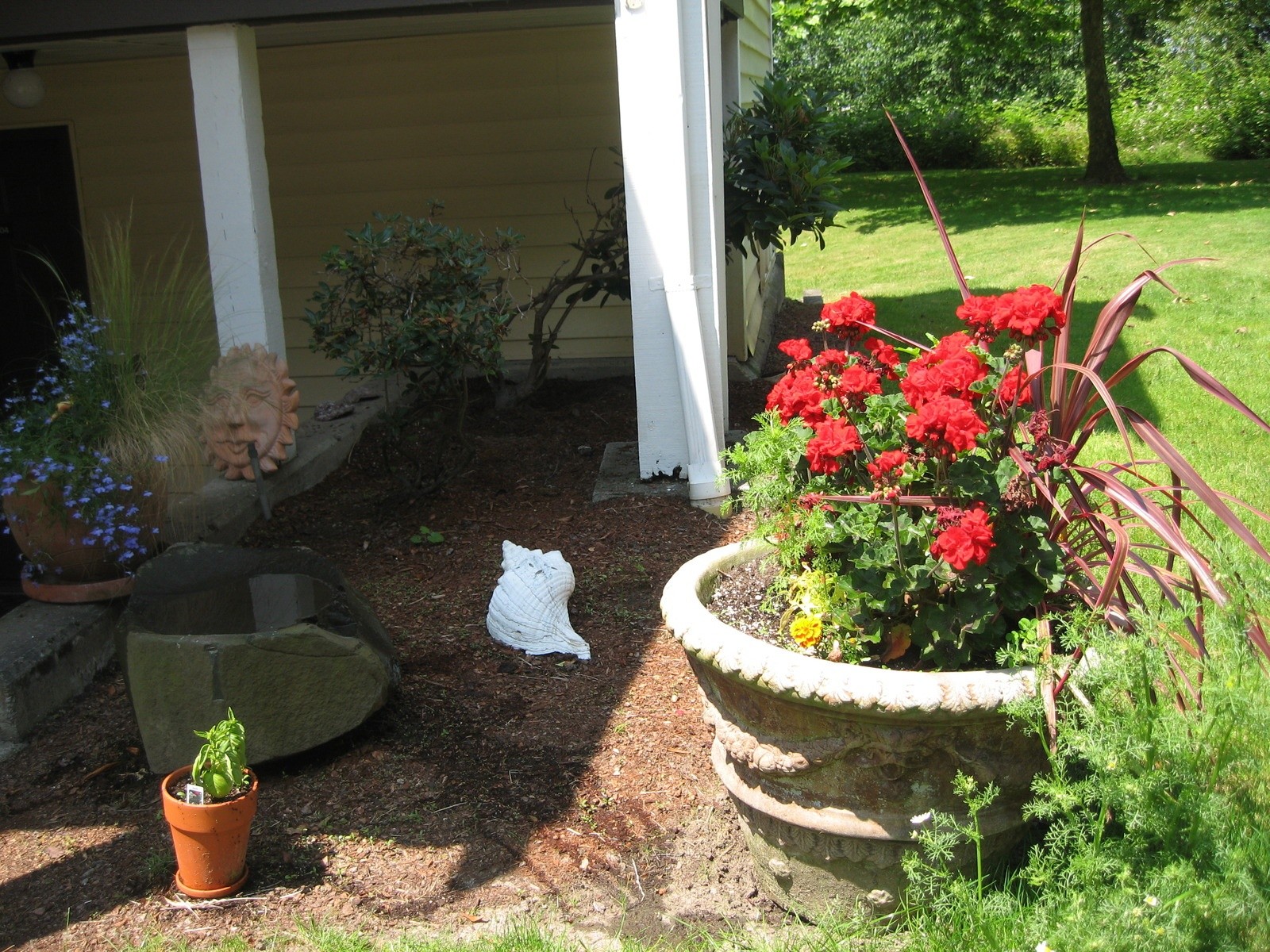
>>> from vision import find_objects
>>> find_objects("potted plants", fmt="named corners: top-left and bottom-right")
top-left (162, 707), bottom-right (258, 898)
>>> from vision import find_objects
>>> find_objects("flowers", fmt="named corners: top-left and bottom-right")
top-left (0, 191), bottom-right (254, 593)
top-left (718, 103), bottom-right (1270, 759)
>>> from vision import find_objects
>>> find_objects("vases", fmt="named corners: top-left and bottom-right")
top-left (2, 478), bottom-right (169, 583)
top-left (656, 539), bottom-right (1103, 937)
